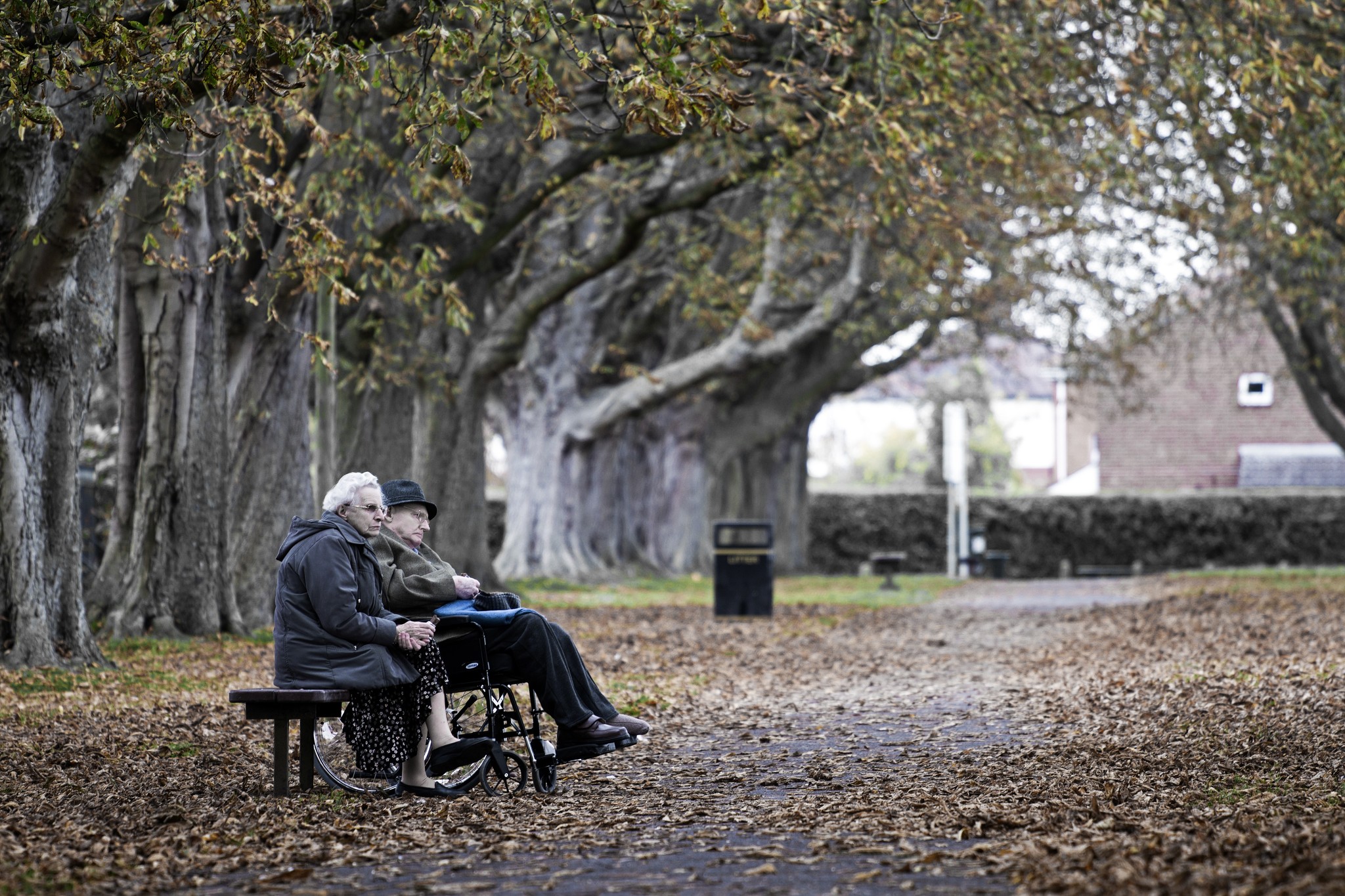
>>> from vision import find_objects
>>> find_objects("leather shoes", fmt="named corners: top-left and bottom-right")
top-left (556, 713), bottom-right (630, 751)
top-left (394, 781), bottom-right (468, 800)
top-left (429, 737), bottom-right (494, 777)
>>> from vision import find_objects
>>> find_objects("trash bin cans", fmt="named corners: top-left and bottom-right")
top-left (711, 519), bottom-right (774, 617)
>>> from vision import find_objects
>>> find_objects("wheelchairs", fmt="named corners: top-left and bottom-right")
top-left (311, 615), bottom-right (638, 803)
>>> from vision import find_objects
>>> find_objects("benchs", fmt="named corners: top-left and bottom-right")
top-left (228, 688), bottom-right (349, 794)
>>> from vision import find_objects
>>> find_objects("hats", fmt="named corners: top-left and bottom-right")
top-left (474, 590), bottom-right (521, 612)
top-left (380, 479), bottom-right (438, 521)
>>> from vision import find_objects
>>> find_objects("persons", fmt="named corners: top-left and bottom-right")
top-left (274, 471), bottom-right (493, 799)
top-left (366, 479), bottom-right (650, 748)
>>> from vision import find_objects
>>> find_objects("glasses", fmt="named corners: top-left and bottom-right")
top-left (400, 503), bottom-right (430, 527)
top-left (344, 503), bottom-right (387, 517)
